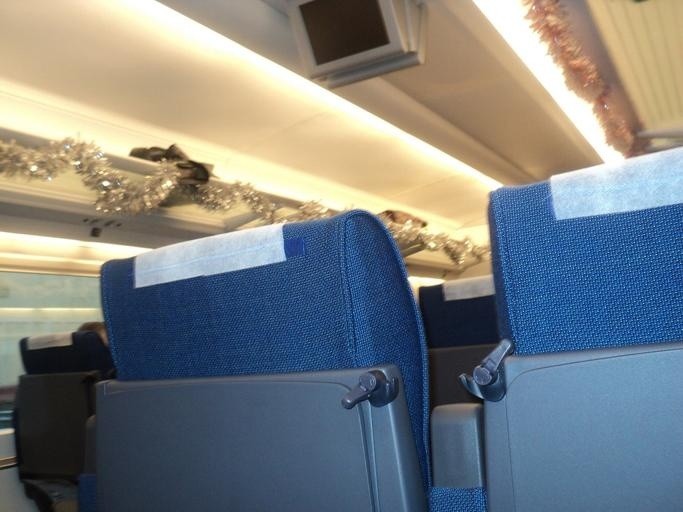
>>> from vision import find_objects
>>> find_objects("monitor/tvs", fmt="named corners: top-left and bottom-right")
top-left (287, 0), bottom-right (429, 89)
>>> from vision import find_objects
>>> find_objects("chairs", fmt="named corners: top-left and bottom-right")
top-left (418, 275), bottom-right (497, 404)
top-left (77, 209), bottom-right (488, 511)
top-left (13, 331), bottom-right (115, 512)
top-left (453, 145), bottom-right (683, 510)
